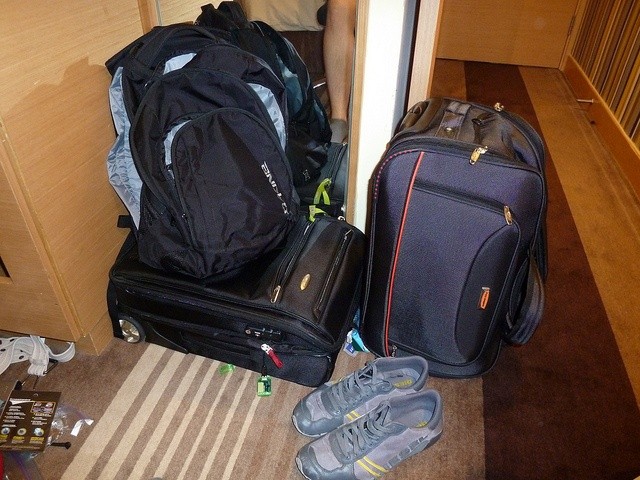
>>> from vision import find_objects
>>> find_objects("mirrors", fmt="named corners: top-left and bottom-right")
top-left (135, 1), bottom-right (369, 224)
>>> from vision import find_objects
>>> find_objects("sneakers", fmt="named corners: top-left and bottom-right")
top-left (292, 356), bottom-right (429, 437)
top-left (295, 390), bottom-right (443, 480)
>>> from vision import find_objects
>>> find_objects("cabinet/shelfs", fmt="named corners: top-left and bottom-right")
top-left (2, 1), bottom-right (144, 360)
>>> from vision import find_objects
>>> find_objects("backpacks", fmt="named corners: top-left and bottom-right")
top-left (194, 2), bottom-right (333, 183)
top-left (105, 23), bottom-right (301, 284)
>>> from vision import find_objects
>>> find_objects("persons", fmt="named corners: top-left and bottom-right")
top-left (242, 0), bottom-right (357, 143)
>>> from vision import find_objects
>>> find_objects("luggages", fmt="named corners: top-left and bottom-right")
top-left (360, 97), bottom-right (546, 379)
top-left (107, 216), bottom-right (364, 388)
top-left (301, 142), bottom-right (348, 216)
